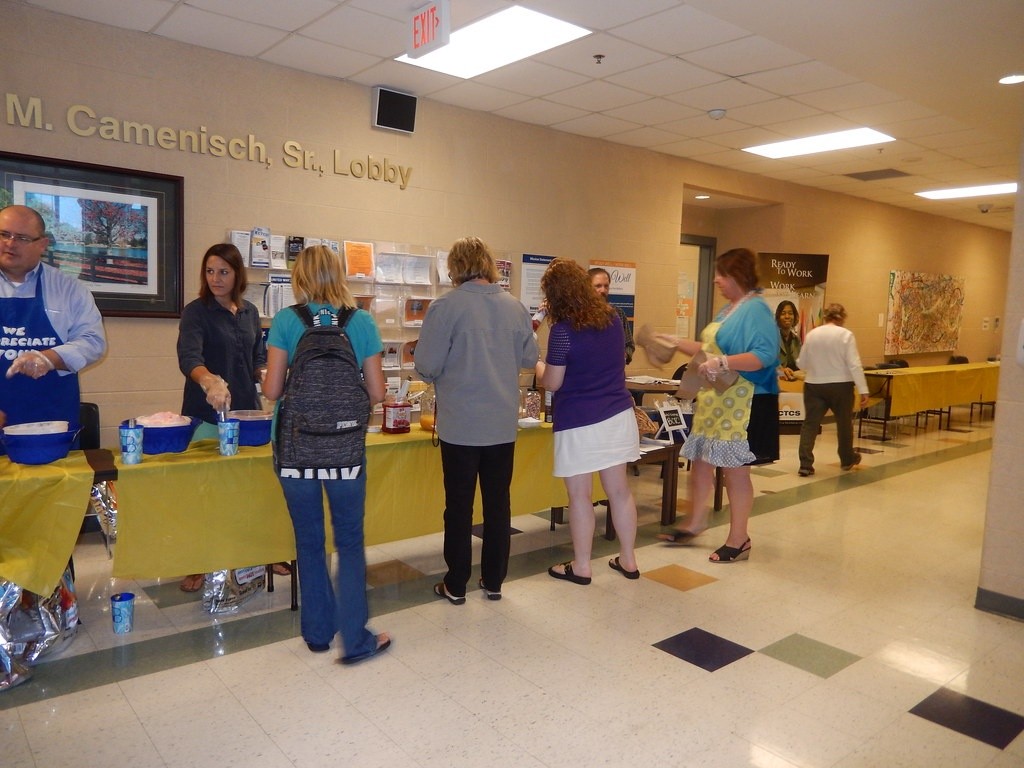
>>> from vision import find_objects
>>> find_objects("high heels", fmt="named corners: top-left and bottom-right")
top-left (657, 528), bottom-right (709, 546)
top-left (709, 538), bottom-right (751, 563)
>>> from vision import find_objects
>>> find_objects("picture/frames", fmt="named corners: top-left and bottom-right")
top-left (0, 149), bottom-right (184, 322)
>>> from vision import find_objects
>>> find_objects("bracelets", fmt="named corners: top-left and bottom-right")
top-left (715, 356), bottom-right (729, 373)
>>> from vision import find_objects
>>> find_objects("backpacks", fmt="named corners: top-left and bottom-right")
top-left (273, 303), bottom-right (370, 481)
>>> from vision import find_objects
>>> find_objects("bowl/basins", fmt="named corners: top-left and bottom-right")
top-left (0, 420), bottom-right (84, 465)
top-left (122, 415), bottom-right (204, 455)
top-left (519, 417), bottom-right (544, 428)
top-left (219, 409), bottom-right (273, 446)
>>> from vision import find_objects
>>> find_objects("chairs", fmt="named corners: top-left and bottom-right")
top-left (888, 359), bottom-right (910, 369)
top-left (950, 356), bottom-right (969, 365)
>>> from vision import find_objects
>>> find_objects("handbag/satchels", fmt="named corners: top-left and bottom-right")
top-left (632, 397), bottom-right (657, 434)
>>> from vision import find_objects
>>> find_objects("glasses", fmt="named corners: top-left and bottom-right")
top-left (0, 231), bottom-right (43, 246)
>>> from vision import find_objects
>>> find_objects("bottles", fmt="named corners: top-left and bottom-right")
top-left (420, 385), bottom-right (435, 431)
top-left (382, 396), bottom-right (410, 435)
top-left (518, 387), bottom-right (553, 423)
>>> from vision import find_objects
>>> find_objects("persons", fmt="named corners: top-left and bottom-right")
top-left (588, 267), bottom-right (634, 364)
top-left (175, 244), bottom-right (290, 591)
top-left (533, 257), bottom-right (642, 586)
top-left (0, 205), bottom-right (105, 453)
top-left (413, 237), bottom-right (540, 604)
top-left (775, 300), bottom-right (802, 382)
top-left (661, 248), bottom-right (780, 563)
top-left (795, 304), bottom-right (868, 476)
top-left (260, 244), bottom-right (391, 663)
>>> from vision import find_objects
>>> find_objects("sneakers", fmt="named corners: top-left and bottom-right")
top-left (798, 465), bottom-right (815, 475)
top-left (841, 452), bottom-right (861, 470)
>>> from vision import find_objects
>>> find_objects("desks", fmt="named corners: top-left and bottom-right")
top-left (858, 362), bottom-right (1002, 440)
top-left (0, 382), bottom-right (724, 613)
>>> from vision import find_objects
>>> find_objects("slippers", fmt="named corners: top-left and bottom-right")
top-left (434, 582), bottom-right (466, 605)
top-left (180, 574), bottom-right (206, 592)
top-left (264, 563), bottom-right (291, 575)
top-left (306, 641), bottom-right (330, 651)
top-left (478, 578), bottom-right (502, 599)
top-left (608, 557), bottom-right (640, 579)
top-left (549, 560), bottom-right (591, 584)
top-left (342, 633), bottom-right (391, 664)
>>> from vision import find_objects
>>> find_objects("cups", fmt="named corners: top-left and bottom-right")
top-left (111, 593), bottom-right (136, 635)
top-left (219, 419), bottom-right (239, 455)
top-left (118, 425), bottom-right (144, 464)
